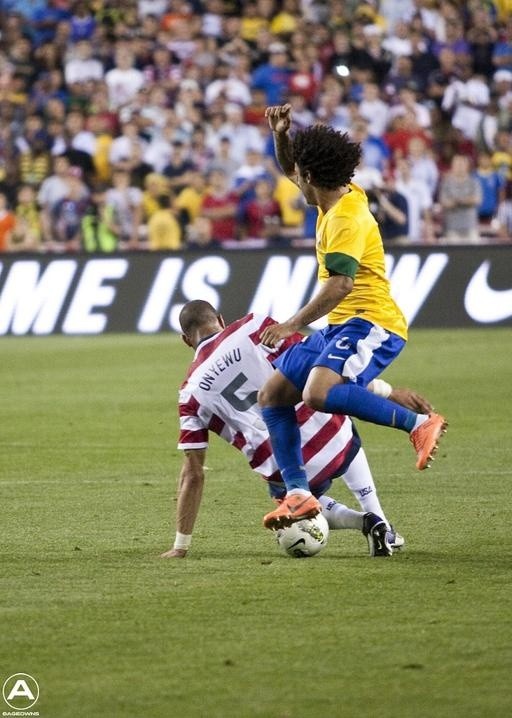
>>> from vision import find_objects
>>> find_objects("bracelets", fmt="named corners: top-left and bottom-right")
top-left (373, 377), bottom-right (393, 399)
top-left (174, 532), bottom-right (193, 551)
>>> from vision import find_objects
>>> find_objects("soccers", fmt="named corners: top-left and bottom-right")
top-left (275, 514), bottom-right (330, 557)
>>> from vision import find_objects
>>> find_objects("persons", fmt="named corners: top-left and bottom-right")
top-left (256, 103), bottom-right (448, 531)
top-left (0, 0), bottom-right (512, 252)
top-left (159, 300), bottom-right (435, 560)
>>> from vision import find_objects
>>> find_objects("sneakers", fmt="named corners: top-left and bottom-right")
top-left (386, 523), bottom-right (404, 547)
top-left (362, 511), bottom-right (393, 557)
top-left (408, 412), bottom-right (449, 471)
top-left (263, 492), bottom-right (324, 532)
